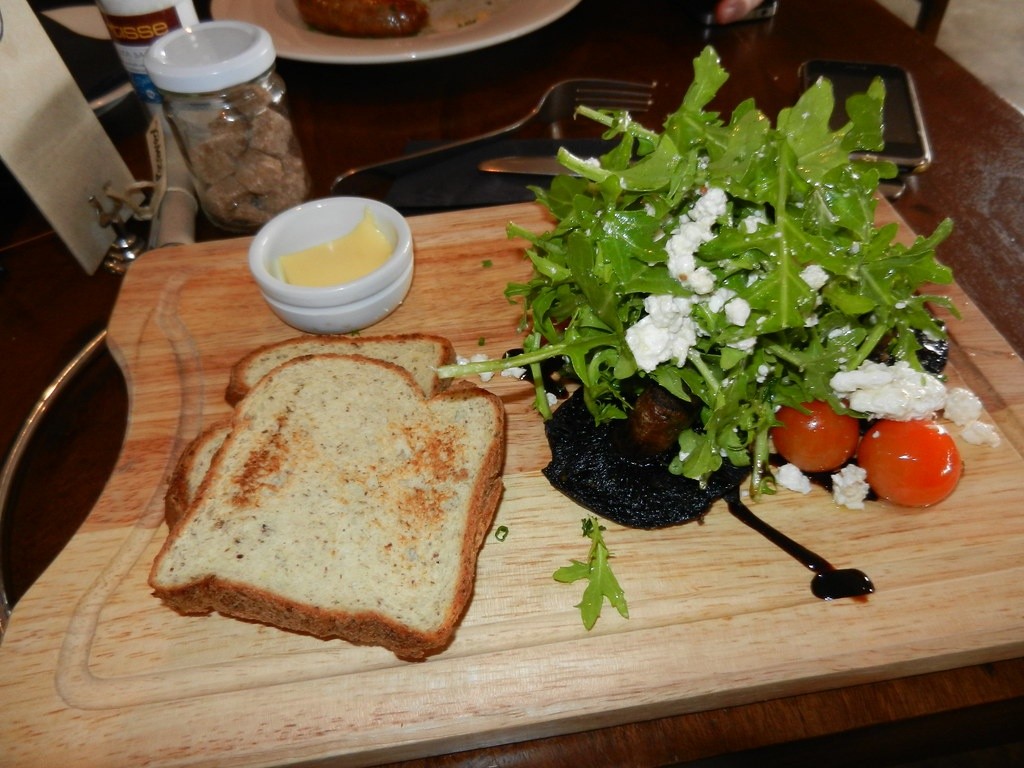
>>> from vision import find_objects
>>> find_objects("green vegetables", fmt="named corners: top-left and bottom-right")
top-left (433, 44), bottom-right (966, 632)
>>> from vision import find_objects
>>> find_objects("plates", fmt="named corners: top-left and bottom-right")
top-left (207, 1), bottom-right (575, 67)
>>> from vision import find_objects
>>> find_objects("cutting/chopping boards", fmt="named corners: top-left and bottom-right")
top-left (0, 191), bottom-right (1022, 765)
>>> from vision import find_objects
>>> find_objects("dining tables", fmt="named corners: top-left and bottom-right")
top-left (1, 1), bottom-right (1024, 766)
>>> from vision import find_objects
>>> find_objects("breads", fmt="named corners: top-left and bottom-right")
top-left (147, 333), bottom-right (507, 662)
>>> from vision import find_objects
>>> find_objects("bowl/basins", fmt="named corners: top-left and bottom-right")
top-left (245, 196), bottom-right (413, 333)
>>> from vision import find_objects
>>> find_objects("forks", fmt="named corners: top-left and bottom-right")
top-left (331, 77), bottom-right (657, 190)
